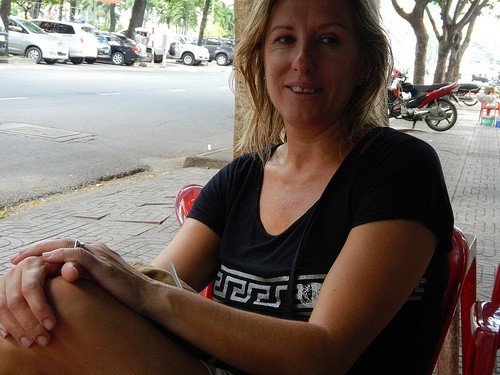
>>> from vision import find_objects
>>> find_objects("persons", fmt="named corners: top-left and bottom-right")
top-left (0, 1), bottom-right (454, 375)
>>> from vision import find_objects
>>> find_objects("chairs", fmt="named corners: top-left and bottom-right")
top-left (478, 102), bottom-right (500, 122)
top-left (174, 183), bottom-right (470, 375)
top-left (470, 264), bottom-right (500, 375)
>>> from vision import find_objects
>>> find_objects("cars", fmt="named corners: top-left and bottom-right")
top-left (111, 32), bottom-right (147, 66)
top-left (99, 31), bottom-right (139, 65)
top-left (28, 18), bottom-right (98, 65)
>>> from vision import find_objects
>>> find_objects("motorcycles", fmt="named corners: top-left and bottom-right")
top-left (449, 83), bottom-right (482, 106)
top-left (385, 64), bottom-right (460, 132)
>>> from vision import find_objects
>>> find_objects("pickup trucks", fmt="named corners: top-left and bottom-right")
top-left (190, 37), bottom-right (236, 66)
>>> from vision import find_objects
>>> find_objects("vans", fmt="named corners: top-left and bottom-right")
top-left (149, 33), bottom-right (210, 66)
top-left (7, 16), bottom-right (70, 64)
top-left (0, 15), bottom-right (9, 56)
top-left (79, 23), bottom-right (111, 63)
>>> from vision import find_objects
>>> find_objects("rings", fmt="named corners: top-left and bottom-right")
top-left (74, 239), bottom-right (85, 248)
